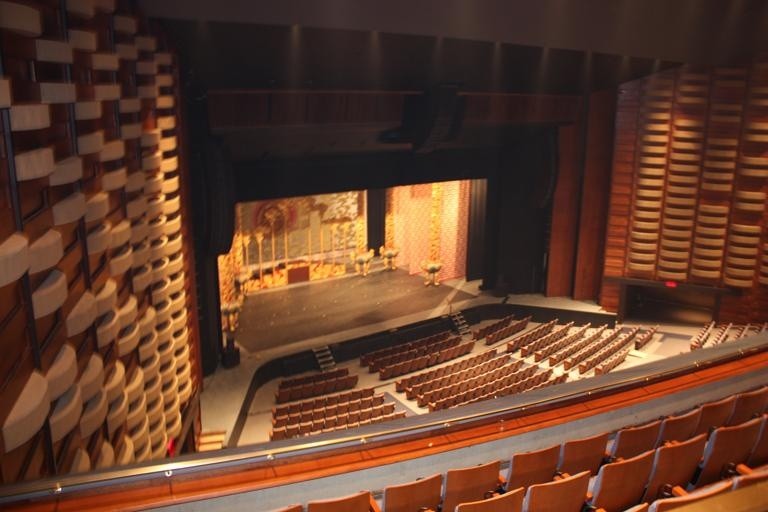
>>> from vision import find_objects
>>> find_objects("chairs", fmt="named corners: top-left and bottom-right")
top-left (690, 320), bottom-right (768, 351)
